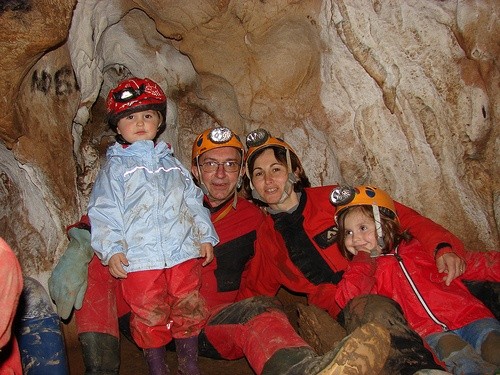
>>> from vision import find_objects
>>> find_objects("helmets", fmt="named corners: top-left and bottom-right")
top-left (191, 128), bottom-right (245, 166)
top-left (105, 77), bottom-right (168, 134)
top-left (244, 136), bottom-right (304, 179)
top-left (334, 183), bottom-right (400, 226)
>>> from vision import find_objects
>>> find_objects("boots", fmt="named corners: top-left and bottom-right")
top-left (259, 320), bottom-right (390, 375)
top-left (173, 336), bottom-right (200, 374)
top-left (17, 313), bottom-right (69, 375)
top-left (80, 332), bottom-right (122, 375)
top-left (145, 345), bottom-right (171, 374)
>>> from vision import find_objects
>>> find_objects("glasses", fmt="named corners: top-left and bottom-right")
top-left (200, 161), bottom-right (244, 173)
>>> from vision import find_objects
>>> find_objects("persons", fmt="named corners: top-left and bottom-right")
top-left (86, 77), bottom-right (221, 375)
top-left (328, 184), bottom-right (500, 375)
top-left (46, 125), bottom-right (500, 375)
top-left (0, 236), bottom-right (69, 375)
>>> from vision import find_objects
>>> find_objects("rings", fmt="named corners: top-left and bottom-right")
top-left (459, 268), bottom-right (464, 272)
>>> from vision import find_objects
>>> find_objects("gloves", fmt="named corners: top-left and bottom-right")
top-left (47, 228), bottom-right (97, 320)
